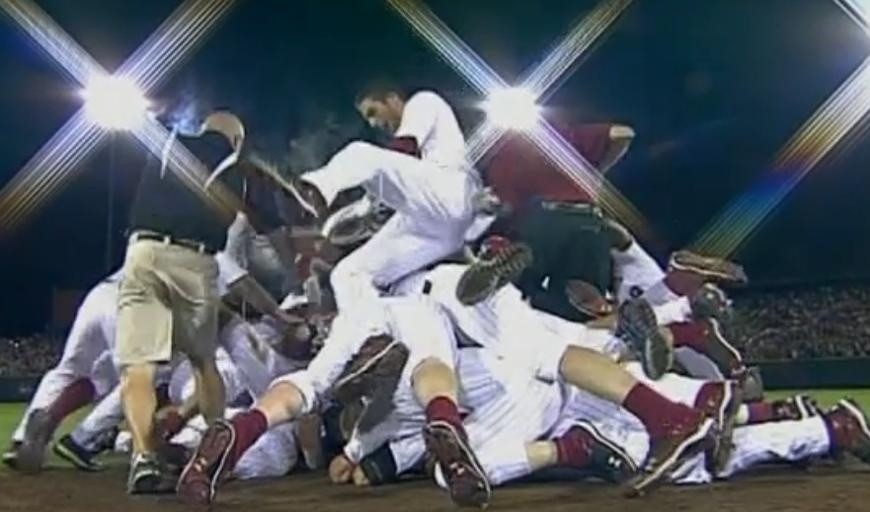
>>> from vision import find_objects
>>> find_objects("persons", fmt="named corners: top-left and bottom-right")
top-left (1, 75), bottom-right (869, 511)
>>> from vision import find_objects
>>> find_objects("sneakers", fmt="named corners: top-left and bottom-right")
top-left (421, 415), bottom-right (491, 512)
top-left (51, 433), bottom-right (108, 472)
top-left (619, 298), bottom-right (669, 383)
top-left (172, 418), bottom-right (236, 510)
top-left (693, 379), bottom-right (741, 474)
top-left (696, 316), bottom-right (743, 376)
top-left (619, 402), bottom-right (716, 499)
top-left (669, 248), bottom-right (749, 286)
top-left (564, 418), bottom-right (639, 485)
top-left (772, 393), bottom-right (870, 465)
top-left (456, 242), bottom-right (536, 308)
top-left (2, 407), bottom-right (55, 476)
